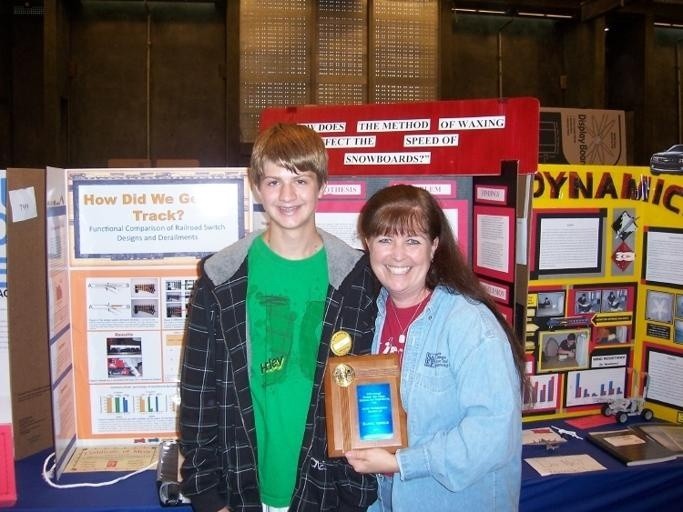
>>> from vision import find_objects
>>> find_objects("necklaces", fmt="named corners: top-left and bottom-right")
top-left (391, 289), bottom-right (427, 344)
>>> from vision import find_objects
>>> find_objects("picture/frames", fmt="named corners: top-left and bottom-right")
top-left (324, 352), bottom-right (408, 458)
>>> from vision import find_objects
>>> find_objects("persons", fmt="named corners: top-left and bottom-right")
top-left (556, 333), bottom-right (577, 359)
top-left (345, 185), bottom-right (530, 512)
top-left (607, 293), bottom-right (615, 309)
top-left (602, 328), bottom-right (620, 344)
top-left (577, 293), bottom-right (586, 312)
top-left (178, 121), bottom-right (382, 512)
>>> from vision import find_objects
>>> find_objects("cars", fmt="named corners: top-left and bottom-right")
top-left (651, 145), bottom-right (682, 176)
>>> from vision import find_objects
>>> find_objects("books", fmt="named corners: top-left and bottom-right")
top-left (588, 429), bottom-right (677, 467)
